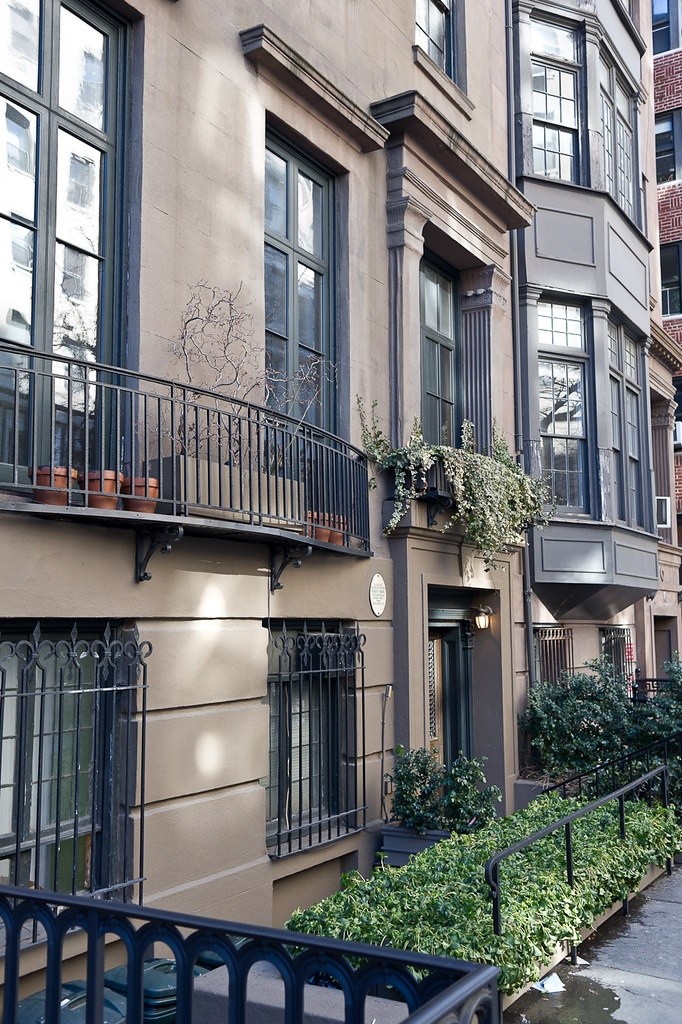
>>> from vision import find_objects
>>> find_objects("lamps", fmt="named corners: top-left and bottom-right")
top-left (475, 602), bottom-right (490, 629)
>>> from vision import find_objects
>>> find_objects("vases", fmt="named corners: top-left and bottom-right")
top-left (117, 476), bottom-right (161, 513)
top-left (27, 465), bottom-right (79, 506)
top-left (75, 470), bottom-right (124, 510)
top-left (295, 509), bottom-right (348, 546)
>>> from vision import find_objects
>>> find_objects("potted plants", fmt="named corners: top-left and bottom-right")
top-left (515, 673), bottom-right (625, 812)
top-left (378, 746), bottom-right (504, 868)
